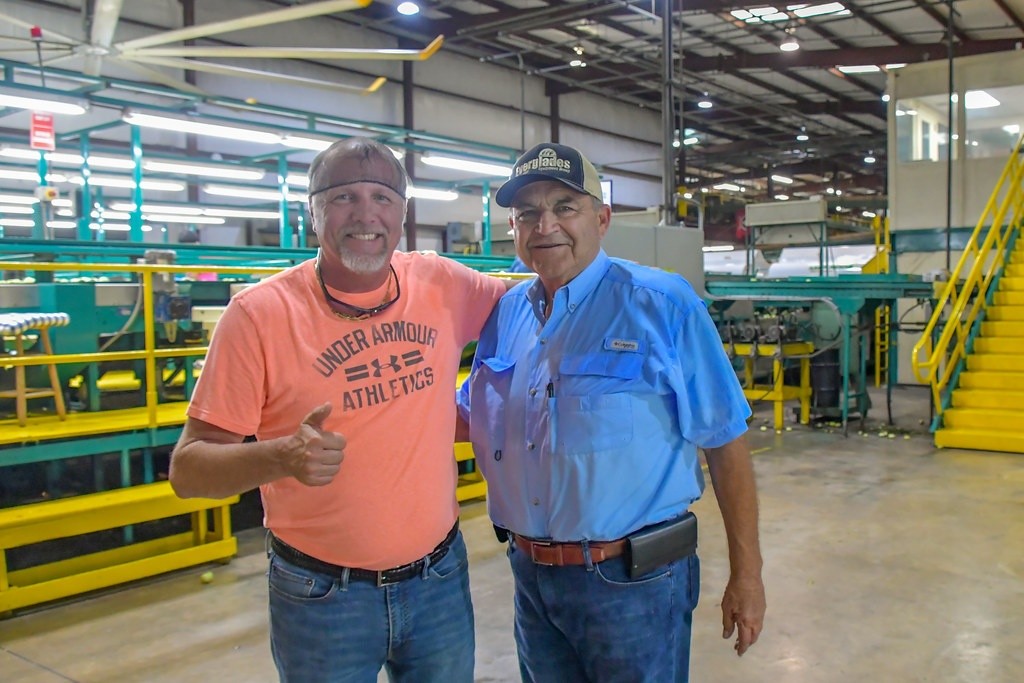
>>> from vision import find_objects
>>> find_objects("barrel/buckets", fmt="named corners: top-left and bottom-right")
top-left (797, 349), bottom-right (840, 406)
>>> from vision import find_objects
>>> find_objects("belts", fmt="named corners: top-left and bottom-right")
top-left (514, 533), bottom-right (630, 565)
top-left (270, 516), bottom-right (460, 583)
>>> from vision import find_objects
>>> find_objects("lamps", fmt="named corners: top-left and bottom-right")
top-left (570, 43), bottom-right (587, 68)
top-left (780, 35), bottom-right (800, 52)
top-left (698, 90), bottom-right (713, 108)
top-left (796, 125), bottom-right (809, 141)
top-left (0, 87), bottom-right (517, 224)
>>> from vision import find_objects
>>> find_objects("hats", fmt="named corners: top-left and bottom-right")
top-left (495, 142), bottom-right (603, 207)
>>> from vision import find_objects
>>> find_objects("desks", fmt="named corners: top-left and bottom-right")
top-left (721, 340), bottom-right (815, 429)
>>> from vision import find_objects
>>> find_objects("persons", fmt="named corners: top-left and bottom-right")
top-left (456, 142), bottom-right (766, 683)
top-left (169, 135), bottom-right (519, 683)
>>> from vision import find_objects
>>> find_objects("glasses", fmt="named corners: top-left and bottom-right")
top-left (318, 263), bottom-right (400, 320)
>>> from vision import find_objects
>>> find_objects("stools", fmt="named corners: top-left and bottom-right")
top-left (0, 312), bottom-right (71, 427)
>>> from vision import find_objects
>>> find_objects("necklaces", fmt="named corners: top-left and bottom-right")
top-left (314, 258), bottom-right (393, 320)
